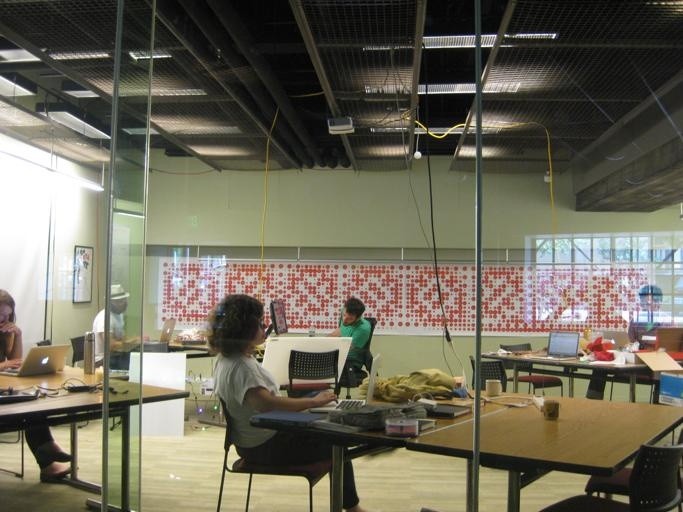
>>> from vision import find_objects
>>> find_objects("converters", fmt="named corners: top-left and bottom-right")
top-left (68, 384), bottom-right (96, 392)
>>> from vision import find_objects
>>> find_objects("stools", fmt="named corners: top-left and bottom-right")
top-left (610, 375), bottom-right (660, 405)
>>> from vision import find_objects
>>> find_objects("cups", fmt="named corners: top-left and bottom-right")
top-left (486, 380), bottom-right (502, 397)
top-left (543, 400), bottom-right (560, 421)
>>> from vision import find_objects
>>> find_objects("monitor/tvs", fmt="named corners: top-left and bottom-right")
top-left (264, 301), bottom-right (288, 337)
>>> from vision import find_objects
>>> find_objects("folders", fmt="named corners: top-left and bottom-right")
top-left (328, 402), bottom-right (426, 430)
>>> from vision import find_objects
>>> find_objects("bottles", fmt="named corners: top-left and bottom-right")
top-left (84, 332), bottom-right (95, 375)
top-left (308, 323), bottom-right (315, 337)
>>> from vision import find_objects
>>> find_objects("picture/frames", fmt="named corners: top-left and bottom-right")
top-left (72, 245), bottom-right (94, 304)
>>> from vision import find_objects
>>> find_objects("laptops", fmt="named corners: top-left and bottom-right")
top-left (0, 343), bottom-right (71, 376)
top-left (309, 353), bottom-right (380, 412)
top-left (141, 318), bottom-right (176, 343)
top-left (532, 331), bottom-right (580, 362)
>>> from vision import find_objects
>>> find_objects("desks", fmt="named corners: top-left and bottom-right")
top-left (262, 336), bottom-right (353, 392)
top-left (249, 390), bottom-right (542, 512)
top-left (128, 350), bottom-right (216, 440)
top-left (0, 365), bottom-right (104, 493)
top-left (0, 380), bottom-right (190, 512)
top-left (481, 351), bottom-right (683, 403)
top-left (406, 395), bottom-right (683, 512)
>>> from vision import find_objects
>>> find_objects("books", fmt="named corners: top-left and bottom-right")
top-left (427, 403), bottom-right (471, 418)
top-left (436, 397), bottom-right (485, 410)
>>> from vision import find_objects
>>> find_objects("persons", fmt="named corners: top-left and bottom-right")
top-left (585, 285), bottom-right (670, 401)
top-left (326, 296), bottom-right (372, 398)
top-left (90, 283), bottom-right (151, 372)
top-left (202, 292), bottom-right (370, 512)
top-left (0, 288), bottom-right (81, 484)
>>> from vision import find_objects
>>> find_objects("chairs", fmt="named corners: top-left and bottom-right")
top-left (500, 343), bottom-right (563, 397)
top-left (343, 317), bottom-right (377, 400)
top-left (216, 398), bottom-right (329, 512)
top-left (538, 444), bottom-right (683, 512)
top-left (280, 350), bottom-right (340, 398)
top-left (470, 356), bottom-right (507, 392)
top-left (0, 430), bottom-right (23, 477)
top-left (585, 427), bottom-right (683, 512)
top-left (70, 336), bottom-right (84, 368)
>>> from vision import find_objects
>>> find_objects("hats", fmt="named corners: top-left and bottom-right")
top-left (103, 283), bottom-right (130, 301)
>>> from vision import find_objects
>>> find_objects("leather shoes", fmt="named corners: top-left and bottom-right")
top-left (35, 451), bottom-right (75, 464)
top-left (41, 465), bottom-right (80, 481)
